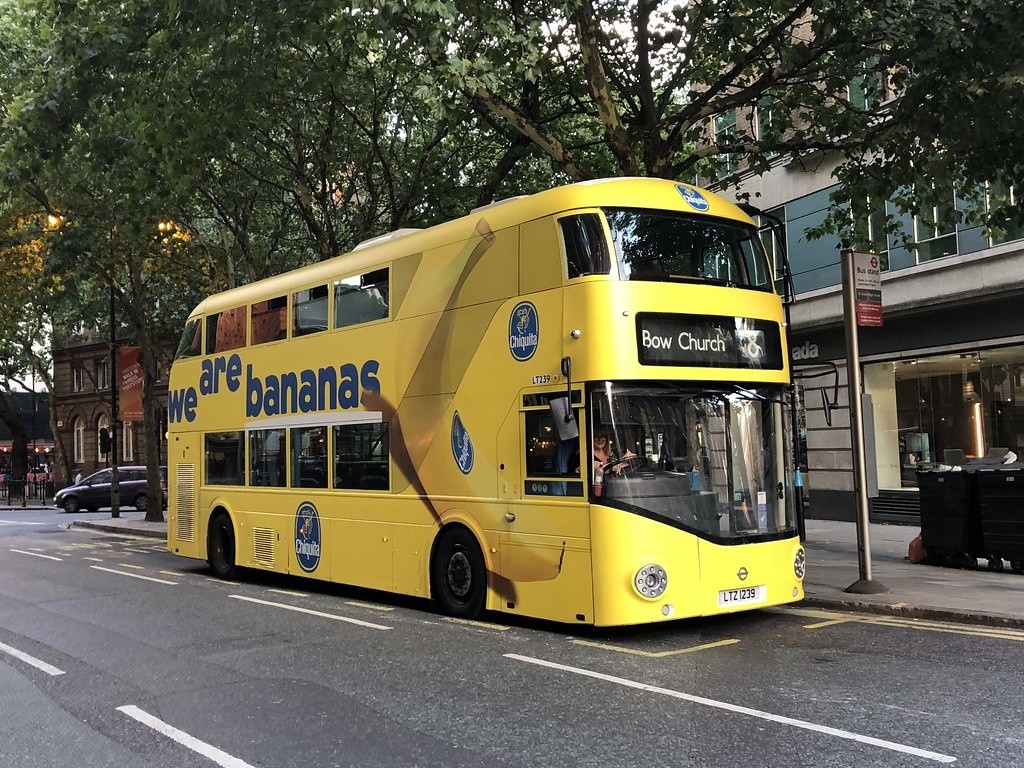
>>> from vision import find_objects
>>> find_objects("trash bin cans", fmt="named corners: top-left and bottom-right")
top-left (914, 457), bottom-right (1024, 574)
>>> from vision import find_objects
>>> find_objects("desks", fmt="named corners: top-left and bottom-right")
top-left (27, 473), bottom-right (49, 499)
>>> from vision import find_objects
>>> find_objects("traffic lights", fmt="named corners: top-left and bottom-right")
top-left (98, 428), bottom-right (109, 453)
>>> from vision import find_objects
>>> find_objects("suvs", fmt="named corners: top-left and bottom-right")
top-left (52, 466), bottom-right (167, 512)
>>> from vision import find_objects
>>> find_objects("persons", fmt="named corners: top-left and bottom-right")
top-left (572, 425), bottom-right (629, 498)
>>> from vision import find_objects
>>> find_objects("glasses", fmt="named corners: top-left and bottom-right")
top-left (594, 435), bottom-right (607, 441)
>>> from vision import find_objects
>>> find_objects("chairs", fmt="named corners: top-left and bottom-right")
top-left (6, 476), bottom-right (27, 507)
top-left (567, 443), bottom-right (620, 496)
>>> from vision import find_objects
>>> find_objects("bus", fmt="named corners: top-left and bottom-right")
top-left (167, 176), bottom-right (841, 629)
